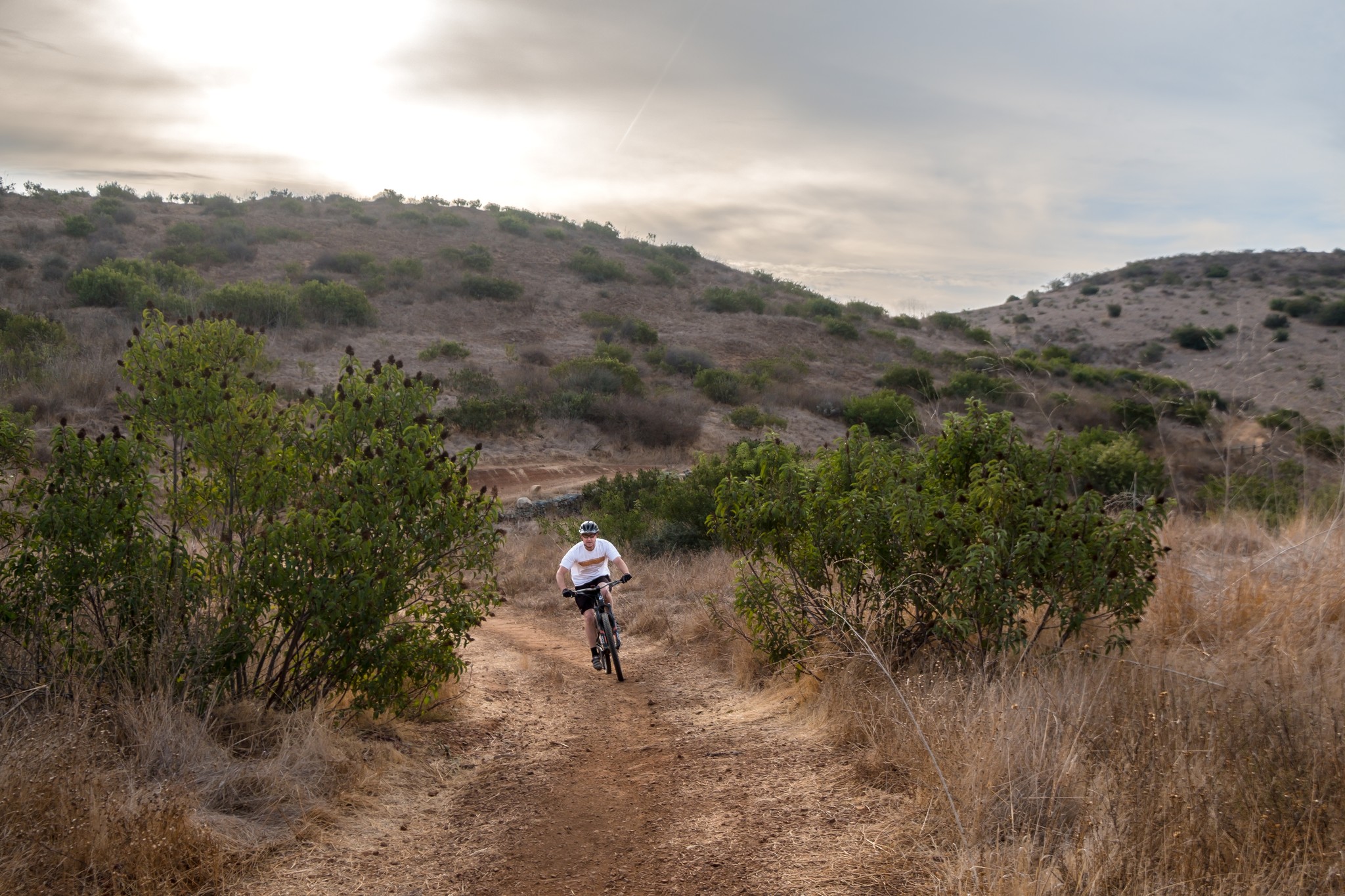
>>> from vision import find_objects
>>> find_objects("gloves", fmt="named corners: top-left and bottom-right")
top-left (562, 588), bottom-right (576, 598)
top-left (619, 573), bottom-right (632, 584)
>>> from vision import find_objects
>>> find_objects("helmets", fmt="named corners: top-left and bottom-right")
top-left (579, 521), bottom-right (599, 534)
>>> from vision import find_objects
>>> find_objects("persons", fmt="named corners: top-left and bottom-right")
top-left (556, 521), bottom-right (632, 670)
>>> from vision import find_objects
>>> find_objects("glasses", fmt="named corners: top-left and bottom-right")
top-left (581, 533), bottom-right (597, 539)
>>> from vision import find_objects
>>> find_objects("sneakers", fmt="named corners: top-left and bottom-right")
top-left (591, 655), bottom-right (603, 670)
top-left (615, 621), bottom-right (620, 629)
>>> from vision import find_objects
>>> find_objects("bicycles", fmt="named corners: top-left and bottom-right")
top-left (572, 578), bottom-right (624, 682)
